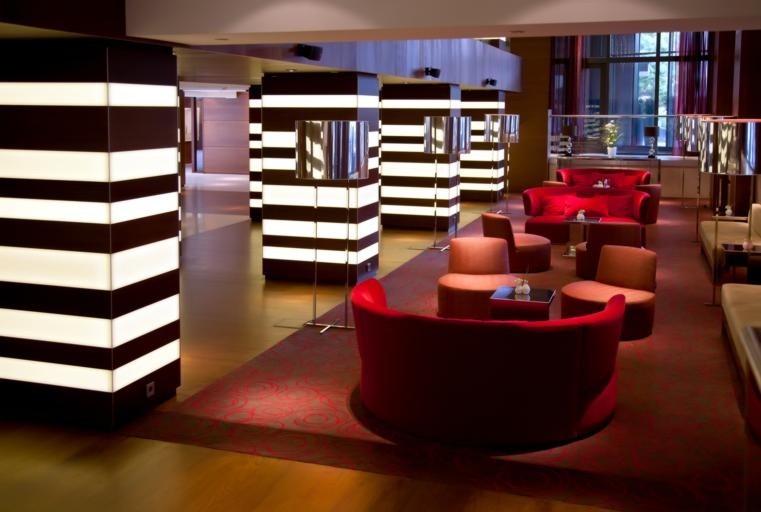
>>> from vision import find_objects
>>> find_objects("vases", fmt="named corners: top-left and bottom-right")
top-left (607, 147), bottom-right (617, 158)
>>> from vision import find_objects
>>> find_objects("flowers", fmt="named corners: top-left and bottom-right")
top-left (604, 120), bottom-right (623, 147)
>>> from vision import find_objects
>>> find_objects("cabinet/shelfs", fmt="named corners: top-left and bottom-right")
top-left (548, 152), bottom-right (659, 185)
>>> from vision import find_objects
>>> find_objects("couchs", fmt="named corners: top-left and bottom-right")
top-left (351, 278), bottom-right (625, 449)
top-left (483, 213), bottom-right (551, 272)
top-left (563, 245), bottom-right (656, 340)
top-left (436, 238), bottom-right (519, 317)
top-left (523, 185), bottom-right (650, 247)
top-left (555, 168), bottom-right (651, 186)
top-left (576, 223), bottom-right (645, 279)
top-left (720, 283), bottom-right (760, 435)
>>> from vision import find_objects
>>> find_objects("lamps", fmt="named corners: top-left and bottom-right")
top-left (423, 116), bottom-right (472, 251)
top-left (484, 113), bottom-right (519, 217)
top-left (677, 113), bottom-right (761, 306)
top-left (292, 119), bottom-right (370, 333)
